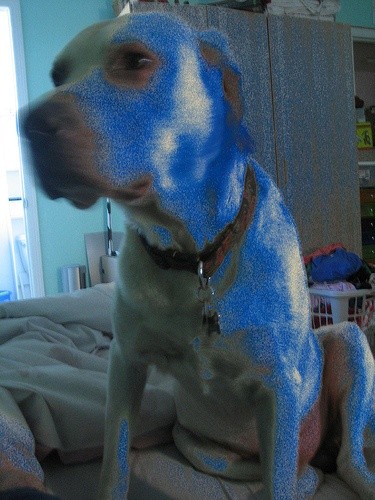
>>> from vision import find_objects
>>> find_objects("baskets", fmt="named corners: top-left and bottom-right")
top-left (306, 287), bottom-right (370, 332)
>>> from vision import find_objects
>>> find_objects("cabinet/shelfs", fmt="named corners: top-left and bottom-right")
top-left (115, 3), bottom-right (375, 269)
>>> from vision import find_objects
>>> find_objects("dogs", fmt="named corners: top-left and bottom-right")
top-left (15, 8), bottom-right (375, 500)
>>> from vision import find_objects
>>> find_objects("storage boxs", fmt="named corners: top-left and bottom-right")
top-left (354, 121), bottom-right (373, 150)
